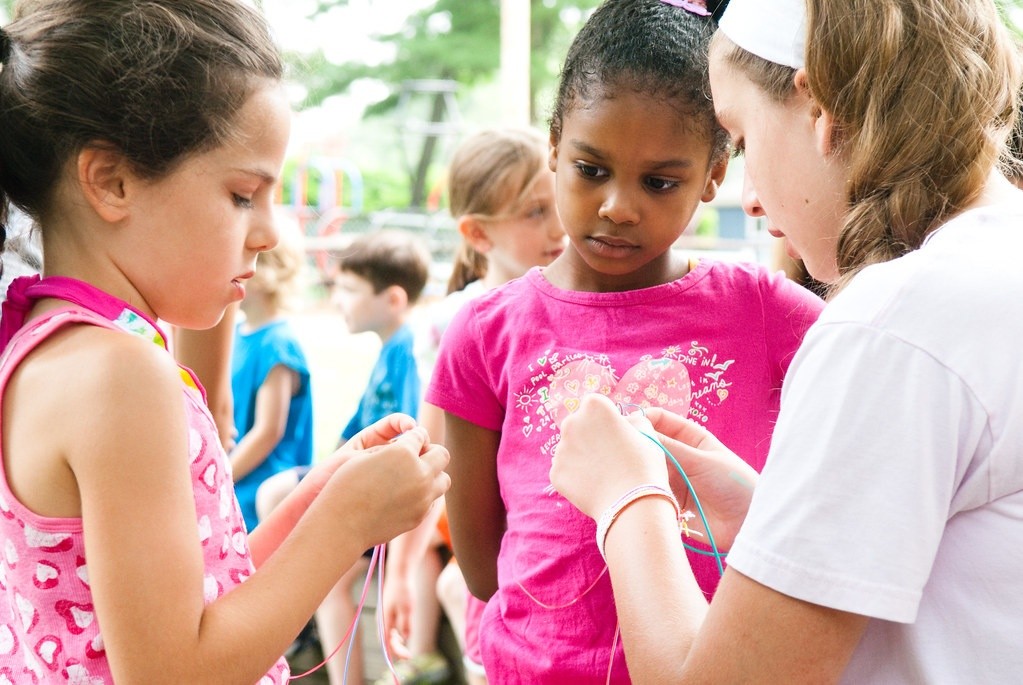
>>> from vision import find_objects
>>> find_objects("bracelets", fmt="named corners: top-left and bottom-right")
top-left (596, 485), bottom-right (680, 564)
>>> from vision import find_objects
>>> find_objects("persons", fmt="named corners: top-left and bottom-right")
top-left (231, 216), bottom-right (331, 685)
top-left (0, 0), bottom-right (450, 685)
top-left (427, 1), bottom-right (825, 685)
top-left (256, 127), bottom-right (571, 685)
top-left (548, 1), bottom-right (1023, 685)
